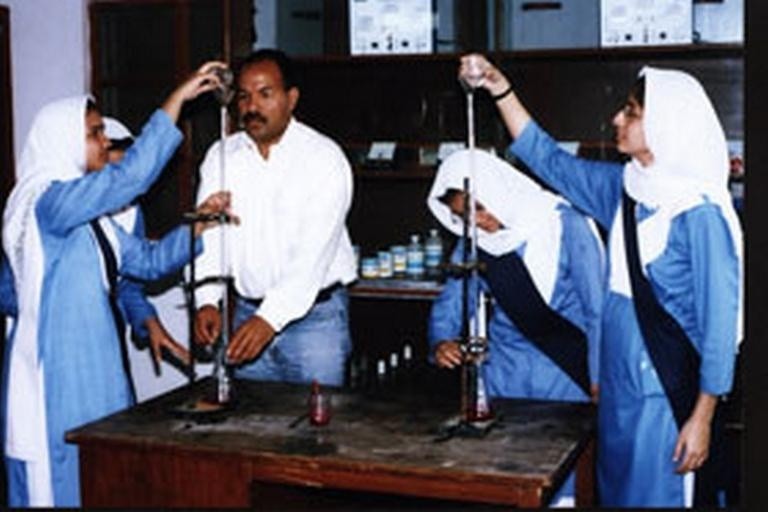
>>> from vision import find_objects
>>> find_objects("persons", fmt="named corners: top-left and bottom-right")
top-left (0, 58), bottom-right (229, 511)
top-left (457, 64), bottom-right (745, 508)
top-left (0, 114), bottom-right (239, 365)
top-left (183, 48), bottom-right (359, 388)
top-left (427, 147), bottom-right (607, 510)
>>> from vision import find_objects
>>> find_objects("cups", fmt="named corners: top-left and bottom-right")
top-left (208, 67), bottom-right (235, 92)
top-left (460, 56), bottom-right (488, 89)
top-left (307, 376), bottom-right (331, 427)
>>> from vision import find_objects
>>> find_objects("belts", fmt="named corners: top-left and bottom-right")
top-left (240, 281), bottom-right (340, 309)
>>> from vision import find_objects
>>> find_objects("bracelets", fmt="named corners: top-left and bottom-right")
top-left (494, 85), bottom-right (514, 103)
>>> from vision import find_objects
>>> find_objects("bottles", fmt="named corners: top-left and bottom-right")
top-left (202, 343), bottom-right (237, 413)
top-left (404, 229), bottom-right (443, 280)
top-left (465, 357), bottom-right (496, 423)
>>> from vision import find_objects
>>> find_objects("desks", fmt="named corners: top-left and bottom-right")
top-left (58, 376), bottom-right (597, 509)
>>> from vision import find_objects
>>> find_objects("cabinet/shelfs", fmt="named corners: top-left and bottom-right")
top-left (87, 2), bottom-right (768, 512)
